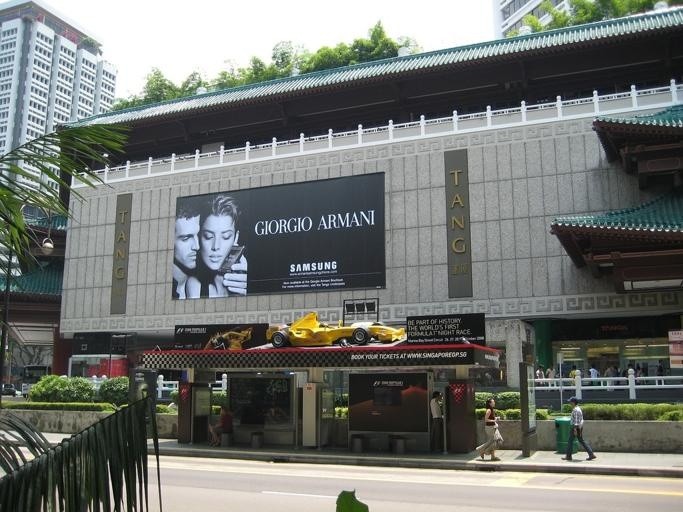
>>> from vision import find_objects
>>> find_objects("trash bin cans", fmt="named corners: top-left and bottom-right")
top-left (554, 418), bottom-right (578, 454)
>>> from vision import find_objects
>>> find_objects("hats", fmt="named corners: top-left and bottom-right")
top-left (566, 396), bottom-right (577, 401)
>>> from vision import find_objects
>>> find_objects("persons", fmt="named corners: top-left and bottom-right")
top-left (479, 398), bottom-right (499, 460)
top-left (562, 396), bottom-right (596, 460)
top-left (209, 332), bottom-right (226, 350)
top-left (429, 391), bottom-right (443, 454)
top-left (536, 360), bottom-right (664, 393)
top-left (186, 195), bottom-right (240, 299)
top-left (172, 207), bottom-right (248, 299)
top-left (209, 405), bottom-right (233, 447)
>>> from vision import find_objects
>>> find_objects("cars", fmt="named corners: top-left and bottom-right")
top-left (0, 383), bottom-right (15, 398)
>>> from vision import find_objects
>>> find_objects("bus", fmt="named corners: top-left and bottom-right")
top-left (18, 364), bottom-right (50, 397)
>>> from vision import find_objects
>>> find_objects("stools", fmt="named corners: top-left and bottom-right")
top-left (219, 431), bottom-right (233, 447)
top-left (387, 435), bottom-right (407, 455)
top-left (350, 434), bottom-right (364, 451)
top-left (250, 432), bottom-right (265, 448)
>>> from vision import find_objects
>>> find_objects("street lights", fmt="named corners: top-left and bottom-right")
top-left (2, 194), bottom-right (55, 387)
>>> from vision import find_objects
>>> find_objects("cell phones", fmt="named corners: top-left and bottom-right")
top-left (218, 245), bottom-right (245, 274)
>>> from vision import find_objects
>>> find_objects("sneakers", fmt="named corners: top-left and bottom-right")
top-left (561, 456), bottom-right (572, 460)
top-left (586, 455), bottom-right (596, 461)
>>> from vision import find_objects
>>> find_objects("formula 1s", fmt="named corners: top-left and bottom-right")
top-left (264, 310), bottom-right (409, 350)
